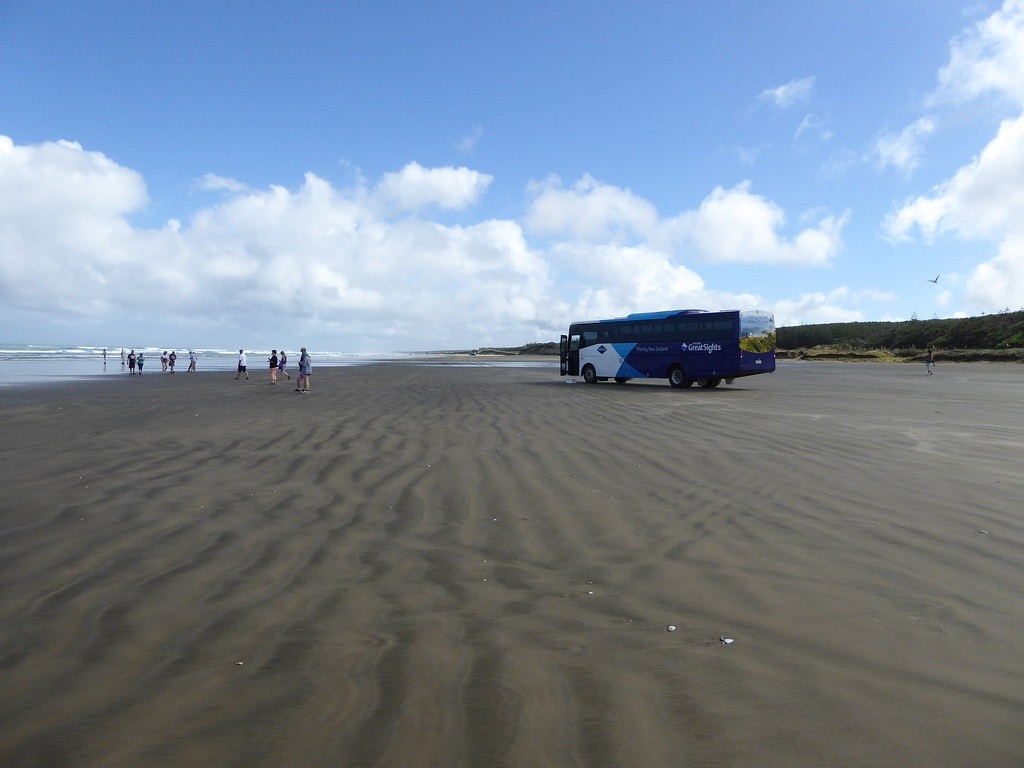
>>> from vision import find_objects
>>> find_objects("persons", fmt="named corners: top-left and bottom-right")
top-left (102, 349), bottom-right (106, 364)
top-left (121, 348), bottom-right (125, 364)
top-left (278, 351), bottom-right (290, 381)
top-left (160, 351), bottom-right (169, 374)
top-left (295, 347), bottom-right (312, 393)
top-left (137, 353), bottom-right (145, 374)
top-left (187, 351), bottom-right (198, 373)
top-left (267, 350), bottom-right (279, 385)
top-left (924, 350), bottom-right (936, 376)
top-left (235, 349), bottom-right (249, 380)
top-left (169, 351), bottom-right (176, 373)
top-left (127, 350), bottom-right (136, 375)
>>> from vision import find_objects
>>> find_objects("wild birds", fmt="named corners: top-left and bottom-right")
top-left (926, 275), bottom-right (940, 283)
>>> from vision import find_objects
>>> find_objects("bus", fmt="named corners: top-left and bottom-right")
top-left (559, 308), bottom-right (778, 389)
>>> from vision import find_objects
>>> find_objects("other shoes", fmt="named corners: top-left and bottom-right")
top-left (294, 389), bottom-right (302, 392)
top-left (302, 389), bottom-right (310, 393)
top-left (288, 375), bottom-right (290, 380)
